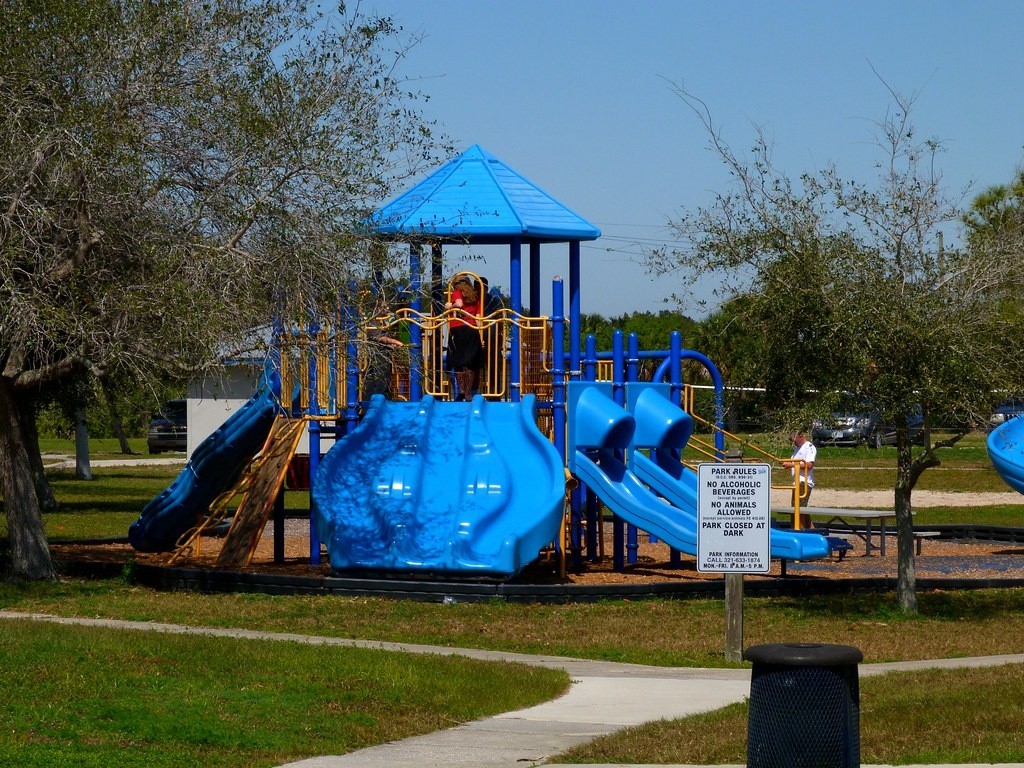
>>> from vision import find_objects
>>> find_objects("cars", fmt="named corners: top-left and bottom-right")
top-left (146, 400), bottom-right (188, 455)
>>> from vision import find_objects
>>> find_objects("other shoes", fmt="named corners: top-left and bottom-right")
top-left (454, 392), bottom-right (467, 401)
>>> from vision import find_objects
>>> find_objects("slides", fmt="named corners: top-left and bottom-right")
top-left (986, 412), bottom-right (1024, 498)
top-left (127, 368), bottom-right (281, 554)
top-left (311, 393), bottom-right (567, 583)
top-left (566, 380), bottom-right (829, 560)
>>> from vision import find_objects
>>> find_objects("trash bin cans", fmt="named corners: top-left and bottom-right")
top-left (741, 641), bottom-right (864, 768)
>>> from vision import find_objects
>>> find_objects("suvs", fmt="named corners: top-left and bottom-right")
top-left (812, 390), bottom-right (928, 449)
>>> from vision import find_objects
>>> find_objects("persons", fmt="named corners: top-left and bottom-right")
top-left (566, 506), bottom-right (587, 547)
top-left (446, 272), bottom-right (501, 402)
top-left (780, 428), bottom-right (817, 530)
top-left (364, 297), bottom-right (405, 402)
